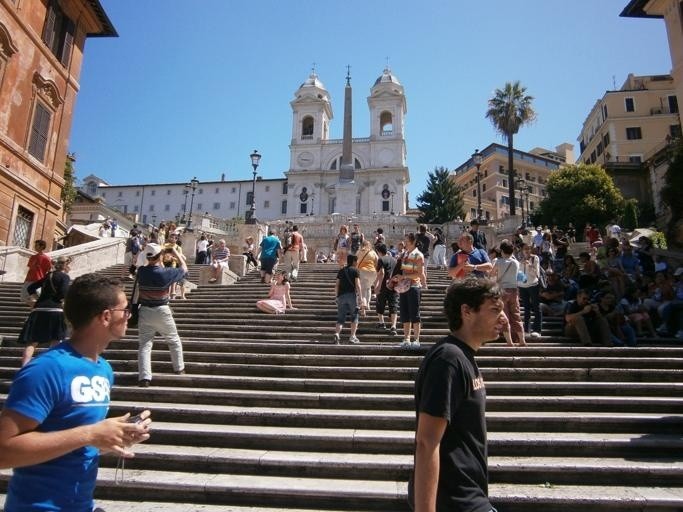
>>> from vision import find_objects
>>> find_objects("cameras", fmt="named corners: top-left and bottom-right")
top-left (122, 415), bottom-right (146, 425)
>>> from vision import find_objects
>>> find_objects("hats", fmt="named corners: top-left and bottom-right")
top-left (673, 267), bottom-right (683, 276)
top-left (434, 228), bottom-right (441, 230)
top-left (145, 243), bottom-right (162, 258)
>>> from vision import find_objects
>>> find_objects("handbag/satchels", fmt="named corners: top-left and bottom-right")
top-left (531, 255), bottom-right (549, 291)
top-left (391, 260), bottom-right (403, 277)
top-left (355, 289), bottom-right (367, 307)
top-left (284, 232), bottom-right (295, 249)
top-left (128, 303), bottom-right (139, 327)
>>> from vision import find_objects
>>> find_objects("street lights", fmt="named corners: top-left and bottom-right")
top-left (246, 150), bottom-right (261, 224)
top-left (514, 177), bottom-right (532, 232)
top-left (180, 176), bottom-right (199, 233)
top-left (470, 149), bottom-right (485, 226)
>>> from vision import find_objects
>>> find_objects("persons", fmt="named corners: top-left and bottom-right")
top-left (243, 220), bottom-right (309, 315)
top-left (98, 216), bottom-right (118, 237)
top-left (127, 214), bottom-right (232, 389)
top-left (448, 223), bottom-right (682, 348)
top-left (19, 239), bottom-right (53, 308)
top-left (317, 225), bottom-right (448, 349)
top-left (1, 270), bottom-right (153, 512)
top-left (16, 254), bottom-right (75, 369)
top-left (405, 275), bottom-right (509, 512)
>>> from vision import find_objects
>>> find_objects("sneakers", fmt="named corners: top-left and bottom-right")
top-left (525, 331), bottom-right (541, 338)
top-left (584, 336), bottom-right (593, 346)
top-left (390, 327), bottom-right (397, 335)
top-left (208, 276), bottom-right (217, 282)
top-left (333, 333), bottom-right (340, 345)
top-left (349, 336), bottom-right (359, 344)
top-left (175, 371), bottom-right (185, 375)
top-left (376, 323), bottom-right (387, 329)
top-left (401, 340), bottom-right (420, 350)
top-left (138, 379), bottom-right (150, 387)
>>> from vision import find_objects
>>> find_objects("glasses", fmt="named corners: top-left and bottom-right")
top-left (109, 303), bottom-right (133, 318)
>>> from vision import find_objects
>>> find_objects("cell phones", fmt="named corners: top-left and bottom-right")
top-left (164, 248), bottom-right (173, 253)
